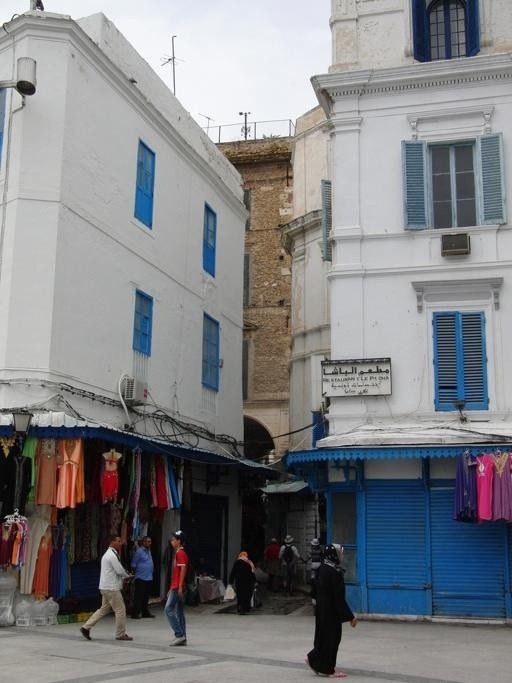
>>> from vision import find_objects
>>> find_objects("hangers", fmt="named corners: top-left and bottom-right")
top-left (4, 508), bottom-right (29, 529)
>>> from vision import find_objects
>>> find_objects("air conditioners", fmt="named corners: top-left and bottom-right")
top-left (440, 231), bottom-right (470, 257)
top-left (124, 377), bottom-right (149, 404)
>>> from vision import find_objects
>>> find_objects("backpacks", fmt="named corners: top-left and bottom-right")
top-left (311, 548), bottom-right (323, 562)
top-left (283, 544), bottom-right (293, 564)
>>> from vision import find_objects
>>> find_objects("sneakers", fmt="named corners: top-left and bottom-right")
top-left (169, 636), bottom-right (187, 646)
top-left (305, 657), bottom-right (348, 678)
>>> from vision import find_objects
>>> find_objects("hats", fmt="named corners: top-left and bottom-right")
top-left (311, 538), bottom-right (320, 546)
top-left (172, 530), bottom-right (185, 544)
top-left (284, 534), bottom-right (295, 542)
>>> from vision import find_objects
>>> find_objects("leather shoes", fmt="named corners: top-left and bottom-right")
top-left (131, 613), bottom-right (156, 618)
top-left (116, 634), bottom-right (133, 640)
top-left (80, 626), bottom-right (91, 640)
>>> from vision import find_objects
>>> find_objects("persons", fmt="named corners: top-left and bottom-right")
top-left (264, 538), bottom-right (281, 576)
top-left (130, 536), bottom-right (155, 619)
top-left (101, 449), bottom-right (122, 504)
top-left (304, 538), bottom-right (324, 606)
top-left (307, 543), bottom-right (356, 677)
top-left (195, 556), bottom-right (214, 576)
top-left (164, 530), bottom-right (189, 646)
top-left (230, 551), bottom-right (256, 615)
top-left (278, 535), bottom-right (306, 598)
top-left (79, 536), bottom-right (134, 640)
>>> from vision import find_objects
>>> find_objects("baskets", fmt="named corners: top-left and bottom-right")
top-left (15, 612), bottom-right (93, 626)
